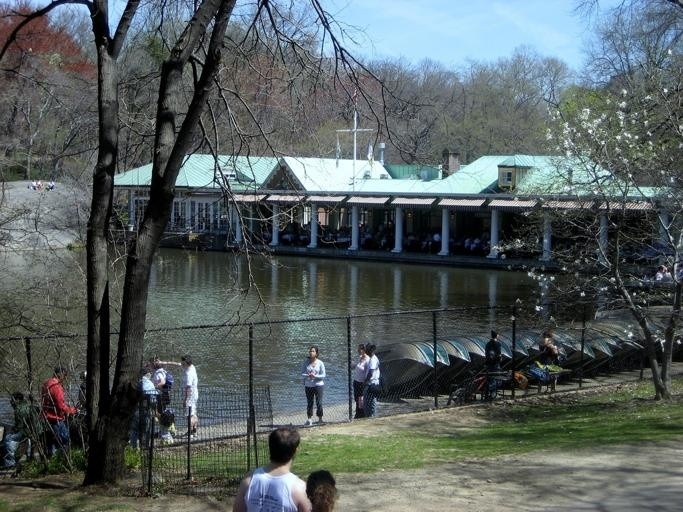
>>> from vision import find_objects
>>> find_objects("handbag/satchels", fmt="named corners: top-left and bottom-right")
top-left (358, 395), bottom-right (364, 409)
top-left (53, 421), bottom-right (69, 449)
top-left (368, 383), bottom-right (383, 394)
top-left (161, 410), bottom-right (174, 427)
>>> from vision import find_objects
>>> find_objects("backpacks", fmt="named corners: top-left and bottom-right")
top-left (164, 371), bottom-right (174, 392)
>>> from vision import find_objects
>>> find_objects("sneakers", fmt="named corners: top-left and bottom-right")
top-left (306, 418), bottom-right (326, 425)
top-left (182, 428), bottom-right (197, 439)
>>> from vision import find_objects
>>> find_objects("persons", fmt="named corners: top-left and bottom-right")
top-left (305, 470), bottom-right (339, 512)
top-left (348, 344), bottom-right (370, 419)
top-left (155, 354), bottom-right (199, 439)
top-left (233, 426), bottom-right (314, 512)
top-left (128, 356), bottom-right (178, 451)
top-left (652, 265), bottom-right (672, 288)
top-left (2, 391), bottom-right (44, 470)
top-left (539, 328), bottom-right (559, 392)
top-left (31, 175), bottom-right (56, 190)
top-left (40, 367), bottom-right (88, 461)
top-left (361, 342), bottom-right (381, 417)
top-left (301, 346), bottom-right (326, 427)
top-left (485, 329), bottom-right (502, 400)
